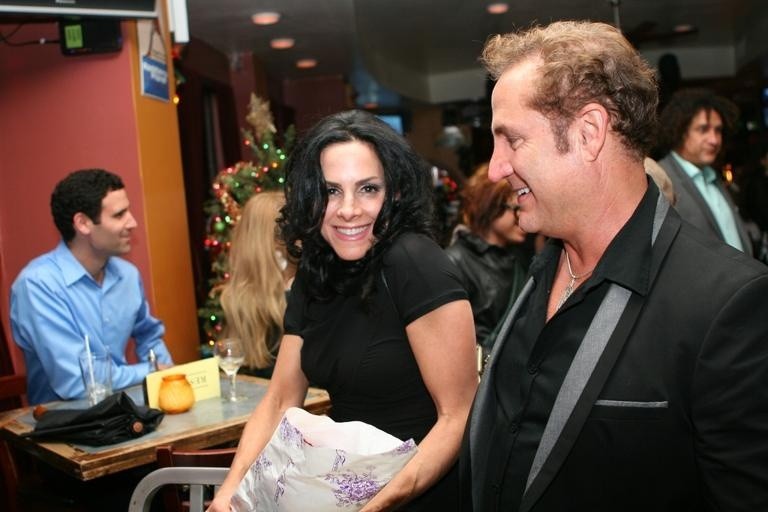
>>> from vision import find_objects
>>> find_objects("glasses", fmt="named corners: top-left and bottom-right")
top-left (502, 203), bottom-right (520, 219)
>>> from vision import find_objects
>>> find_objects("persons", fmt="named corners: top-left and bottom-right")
top-left (4, 164), bottom-right (213, 512)
top-left (445, 15), bottom-right (768, 511)
top-left (436, 161), bottom-right (534, 385)
top-left (648, 86), bottom-right (754, 260)
top-left (203, 186), bottom-right (304, 382)
top-left (198, 105), bottom-right (476, 511)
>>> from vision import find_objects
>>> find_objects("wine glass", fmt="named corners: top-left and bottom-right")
top-left (213, 337), bottom-right (247, 403)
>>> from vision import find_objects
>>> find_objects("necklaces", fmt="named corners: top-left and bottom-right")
top-left (554, 249), bottom-right (597, 314)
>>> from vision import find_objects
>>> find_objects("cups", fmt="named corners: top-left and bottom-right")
top-left (80, 352), bottom-right (114, 406)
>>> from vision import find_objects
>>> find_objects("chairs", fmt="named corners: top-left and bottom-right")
top-left (2, 378), bottom-right (26, 510)
top-left (128, 447), bottom-right (234, 512)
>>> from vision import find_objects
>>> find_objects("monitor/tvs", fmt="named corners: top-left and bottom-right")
top-left (367, 106), bottom-right (412, 138)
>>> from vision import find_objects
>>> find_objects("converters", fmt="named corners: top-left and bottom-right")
top-left (55, 17), bottom-right (124, 57)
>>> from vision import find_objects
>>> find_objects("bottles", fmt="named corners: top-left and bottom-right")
top-left (158, 373), bottom-right (195, 414)
